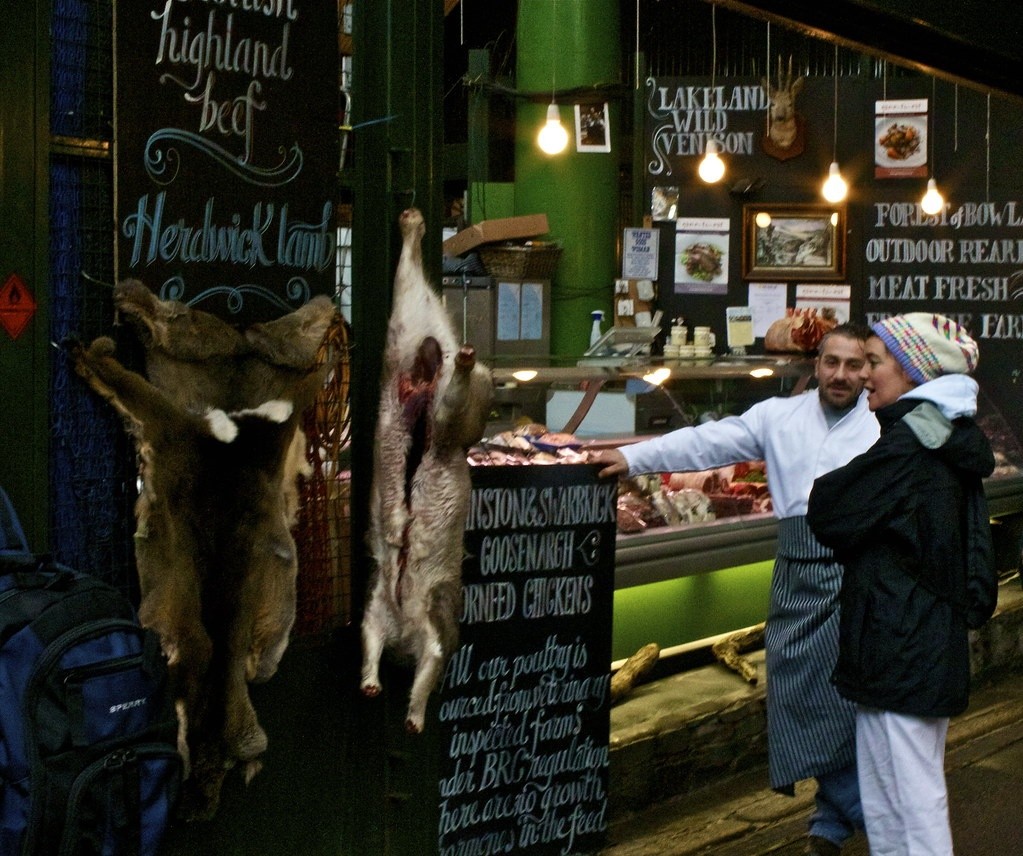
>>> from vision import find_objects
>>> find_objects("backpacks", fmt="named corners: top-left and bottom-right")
top-left (1, 488), bottom-right (185, 855)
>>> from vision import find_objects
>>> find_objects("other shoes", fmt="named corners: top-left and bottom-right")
top-left (804, 837), bottom-right (841, 856)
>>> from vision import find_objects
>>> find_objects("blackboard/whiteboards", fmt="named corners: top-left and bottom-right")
top-left (431, 463), bottom-right (620, 856)
top-left (114, 0), bottom-right (341, 333)
top-left (845, 196), bottom-right (1023, 380)
top-left (645, 75), bottom-right (769, 184)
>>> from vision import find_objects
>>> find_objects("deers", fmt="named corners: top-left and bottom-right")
top-left (760, 54), bottom-right (805, 150)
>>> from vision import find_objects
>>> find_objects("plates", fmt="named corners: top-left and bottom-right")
top-left (875, 115), bottom-right (927, 167)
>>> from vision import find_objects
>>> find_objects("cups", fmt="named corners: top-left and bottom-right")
top-left (669, 473), bottom-right (714, 497)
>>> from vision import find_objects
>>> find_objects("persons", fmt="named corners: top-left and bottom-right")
top-left (806, 310), bottom-right (996, 856)
top-left (584, 322), bottom-right (866, 856)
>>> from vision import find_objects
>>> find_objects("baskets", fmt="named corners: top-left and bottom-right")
top-left (478, 243), bottom-right (563, 281)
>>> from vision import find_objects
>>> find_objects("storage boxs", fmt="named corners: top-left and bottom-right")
top-left (442, 213), bottom-right (551, 257)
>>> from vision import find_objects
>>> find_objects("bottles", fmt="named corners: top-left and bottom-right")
top-left (663, 324), bottom-right (715, 357)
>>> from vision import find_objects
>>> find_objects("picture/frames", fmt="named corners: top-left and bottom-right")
top-left (741, 203), bottom-right (847, 280)
top-left (572, 104), bottom-right (612, 155)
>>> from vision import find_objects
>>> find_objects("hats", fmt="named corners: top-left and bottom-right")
top-left (872, 311), bottom-right (980, 383)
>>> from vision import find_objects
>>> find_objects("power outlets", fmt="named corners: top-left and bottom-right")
top-left (614, 279), bottom-right (629, 295)
top-left (618, 299), bottom-right (634, 317)
